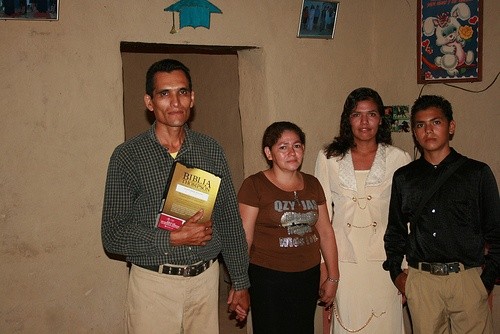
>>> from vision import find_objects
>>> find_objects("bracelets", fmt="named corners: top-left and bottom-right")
top-left (327, 277), bottom-right (339, 283)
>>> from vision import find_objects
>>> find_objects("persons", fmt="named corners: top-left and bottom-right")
top-left (383, 95), bottom-right (500, 334)
top-left (100, 59), bottom-right (251, 334)
top-left (227, 121), bottom-right (340, 334)
top-left (301, 4), bottom-right (335, 32)
top-left (315, 88), bottom-right (413, 334)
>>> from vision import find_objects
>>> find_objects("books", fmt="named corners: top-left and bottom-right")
top-left (155, 160), bottom-right (221, 231)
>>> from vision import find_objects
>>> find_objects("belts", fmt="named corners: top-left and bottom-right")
top-left (133, 258), bottom-right (217, 277)
top-left (408, 261), bottom-right (473, 275)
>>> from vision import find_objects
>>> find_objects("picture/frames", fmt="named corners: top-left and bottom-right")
top-left (296, 0), bottom-right (340, 40)
top-left (0, 0), bottom-right (60, 21)
top-left (416, 0), bottom-right (483, 85)
